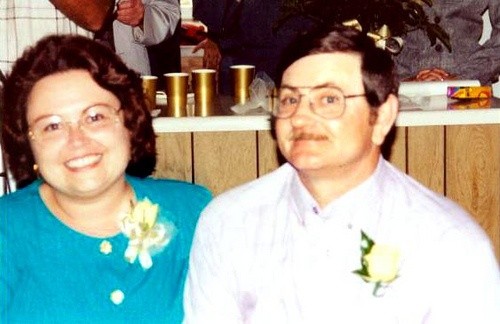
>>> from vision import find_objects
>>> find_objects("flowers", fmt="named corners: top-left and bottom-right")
top-left (351, 229), bottom-right (402, 298)
top-left (117, 196), bottom-right (167, 270)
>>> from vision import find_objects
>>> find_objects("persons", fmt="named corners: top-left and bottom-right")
top-left (183, 25), bottom-right (500, 324)
top-left (0, 34), bottom-right (217, 324)
top-left (48, 0), bottom-right (181, 91)
top-left (391, 0), bottom-right (500, 86)
top-left (192, 0), bottom-right (315, 97)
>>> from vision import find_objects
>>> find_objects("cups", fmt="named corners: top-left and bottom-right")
top-left (230, 65), bottom-right (255, 98)
top-left (163, 73), bottom-right (189, 106)
top-left (193, 69), bottom-right (217, 104)
top-left (140, 75), bottom-right (159, 110)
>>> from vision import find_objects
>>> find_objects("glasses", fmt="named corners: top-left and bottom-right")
top-left (25, 105), bottom-right (123, 143)
top-left (268, 86), bottom-right (368, 119)
